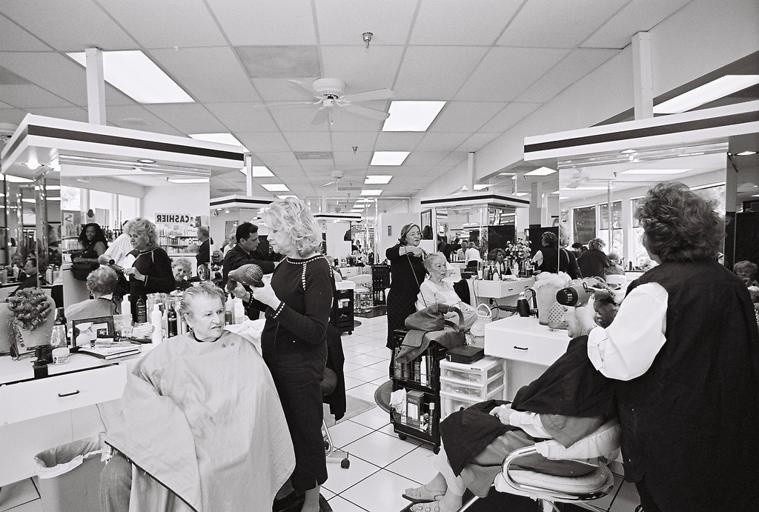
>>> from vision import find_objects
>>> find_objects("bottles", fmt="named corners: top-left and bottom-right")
top-left (477, 257), bottom-right (539, 282)
top-left (50, 307), bottom-right (69, 348)
top-left (101, 225), bottom-right (124, 242)
top-left (118, 289), bottom-right (188, 346)
top-left (224, 290), bottom-right (246, 328)
top-left (156, 226), bottom-right (201, 255)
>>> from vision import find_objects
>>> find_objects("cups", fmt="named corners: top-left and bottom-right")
top-left (52, 346), bottom-right (71, 364)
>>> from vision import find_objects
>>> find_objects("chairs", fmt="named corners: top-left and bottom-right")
top-left (405, 304), bottom-right (466, 389)
top-left (455, 418), bottom-right (620, 512)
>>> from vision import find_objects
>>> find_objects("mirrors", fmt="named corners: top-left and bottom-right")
top-left (559, 156), bottom-right (726, 282)
top-left (435, 208), bottom-right (488, 264)
top-left (60, 165), bottom-right (211, 274)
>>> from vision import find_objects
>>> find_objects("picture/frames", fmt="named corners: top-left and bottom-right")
top-left (421, 209), bottom-right (431, 231)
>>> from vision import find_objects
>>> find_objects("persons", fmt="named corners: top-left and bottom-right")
top-left (530, 232), bottom-right (558, 273)
top-left (351, 240), bottom-right (362, 252)
top-left (100, 282), bottom-right (297, 512)
top-left (221, 196), bottom-right (334, 512)
top-left (404, 277), bottom-right (620, 512)
top-left (384, 222), bottom-right (482, 380)
top-left (488, 248), bottom-right (506, 267)
top-left (565, 184), bottom-right (759, 512)
top-left (10, 258), bottom-right (37, 297)
top-left (11, 238), bottom-right (27, 281)
top-left (63, 217), bottom-right (211, 320)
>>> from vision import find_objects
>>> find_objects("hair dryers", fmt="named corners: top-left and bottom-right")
top-left (557, 282), bottom-right (610, 311)
top-left (97, 253), bottom-right (116, 266)
top-left (226, 263), bottom-right (265, 288)
top-left (499, 290), bottom-right (532, 317)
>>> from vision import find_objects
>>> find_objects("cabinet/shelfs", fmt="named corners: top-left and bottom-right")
top-left (436, 356), bottom-right (510, 454)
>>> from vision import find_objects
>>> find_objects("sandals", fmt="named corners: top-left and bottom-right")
top-left (407, 502), bottom-right (463, 512)
top-left (400, 485), bottom-right (445, 500)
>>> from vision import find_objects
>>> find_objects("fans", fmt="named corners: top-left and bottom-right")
top-left (254, 77), bottom-right (394, 121)
top-left (319, 169), bottom-right (366, 188)
